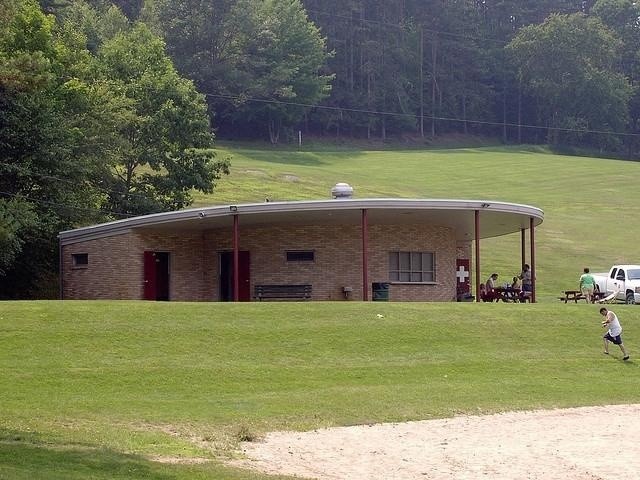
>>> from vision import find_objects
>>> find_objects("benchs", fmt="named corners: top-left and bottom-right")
top-left (560, 290), bottom-right (603, 304)
top-left (493, 286), bottom-right (532, 303)
top-left (253, 284), bottom-right (313, 302)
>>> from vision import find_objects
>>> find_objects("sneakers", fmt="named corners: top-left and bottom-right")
top-left (603, 350), bottom-right (610, 356)
top-left (622, 355), bottom-right (631, 361)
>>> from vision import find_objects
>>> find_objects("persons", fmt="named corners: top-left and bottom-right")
top-left (480, 283), bottom-right (488, 302)
top-left (511, 277), bottom-right (521, 297)
top-left (485, 273), bottom-right (515, 303)
top-left (517, 264), bottom-right (532, 292)
top-left (579, 268), bottom-right (598, 305)
top-left (599, 307), bottom-right (631, 360)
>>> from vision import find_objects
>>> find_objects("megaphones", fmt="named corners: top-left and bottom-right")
top-left (198, 212), bottom-right (205, 220)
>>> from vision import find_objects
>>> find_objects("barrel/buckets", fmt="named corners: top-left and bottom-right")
top-left (372, 282), bottom-right (389, 300)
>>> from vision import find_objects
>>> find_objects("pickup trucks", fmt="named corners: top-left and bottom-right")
top-left (591, 265), bottom-right (640, 305)
